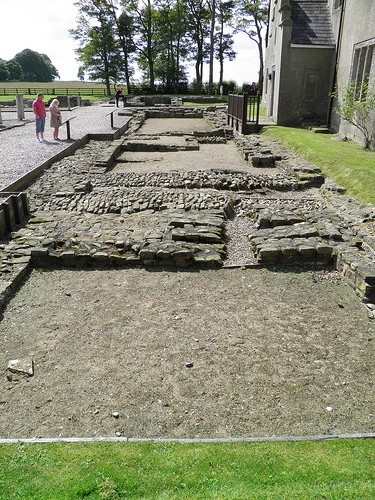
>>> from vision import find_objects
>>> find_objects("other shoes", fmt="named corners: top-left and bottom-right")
top-left (37, 139), bottom-right (43, 143)
top-left (54, 138), bottom-right (60, 140)
top-left (42, 139), bottom-right (48, 142)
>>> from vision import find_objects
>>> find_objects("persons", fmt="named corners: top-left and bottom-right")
top-left (115, 88), bottom-right (125, 107)
top-left (32, 92), bottom-right (46, 142)
top-left (49, 99), bottom-right (62, 140)
top-left (218, 79), bottom-right (259, 100)
